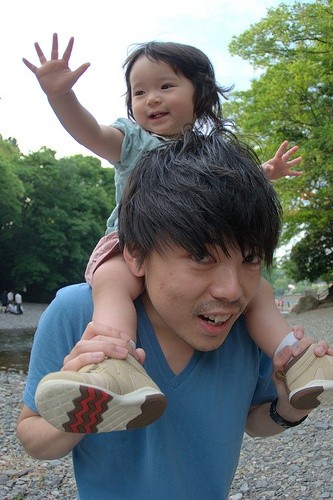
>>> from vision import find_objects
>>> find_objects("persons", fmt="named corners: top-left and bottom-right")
top-left (15, 122), bottom-right (333, 500)
top-left (1, 291), bottom-right (23, 315)
top-left (21, 32), bottom-right (333, 433)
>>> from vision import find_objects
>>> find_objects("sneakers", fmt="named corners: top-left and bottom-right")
top-left (274, 342), bottom-right (333, 410)
top-left (34, 353), bottom-right (167, 434)
